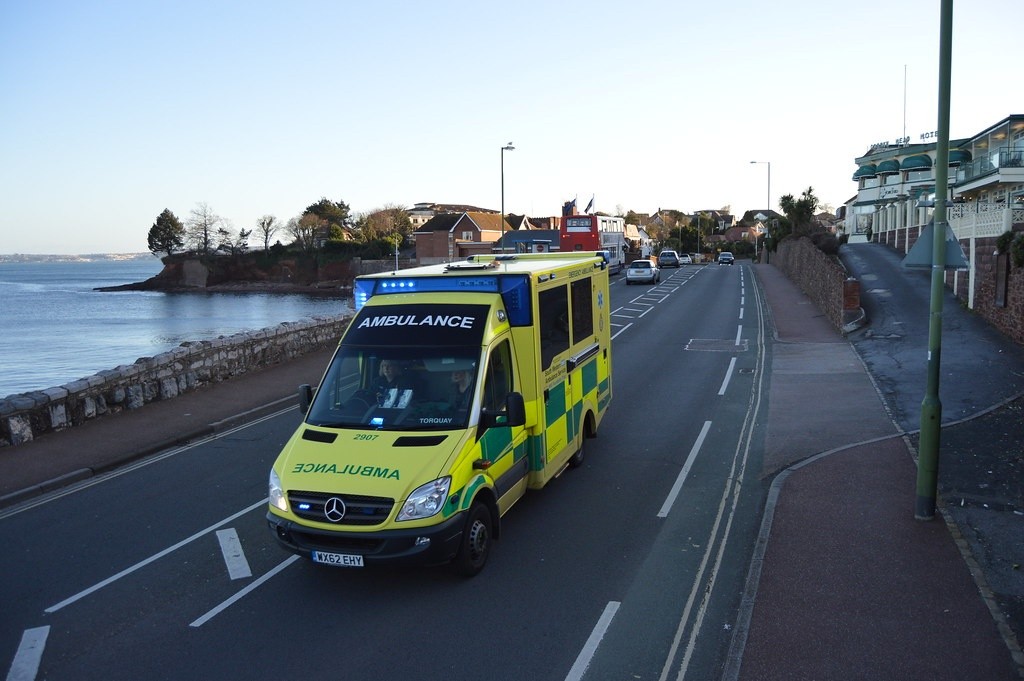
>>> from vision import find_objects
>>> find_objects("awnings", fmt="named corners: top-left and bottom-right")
top-left (852, 150), bottom-right (972, 181)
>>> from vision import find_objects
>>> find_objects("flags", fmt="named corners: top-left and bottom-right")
top-left (564, 199), bottom-right (575, 211)
top-left (584, 199), bottom-right (593, 214)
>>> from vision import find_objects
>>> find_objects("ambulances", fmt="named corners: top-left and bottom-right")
top-left (262, 249), bottom-right (615, 582)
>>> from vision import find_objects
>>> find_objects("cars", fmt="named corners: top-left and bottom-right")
top-left (658, 250), bottom-right (681, 268)
top-left (718, 252), bottom-right (735, 266)
top-left (679, 254), bottom-right (692, 265)
top-left (626, 259), bottom-right (662, 285)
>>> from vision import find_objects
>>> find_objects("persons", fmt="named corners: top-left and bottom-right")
top-left (447, 370), bottom-right (486, 410)
top-left (374, 360), bottom-right (425, 409)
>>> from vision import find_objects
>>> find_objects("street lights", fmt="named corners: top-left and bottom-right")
top-left (750, 160), bottom-right (770, 243)
top-left (500, 145), bottom-right (516, 254)
